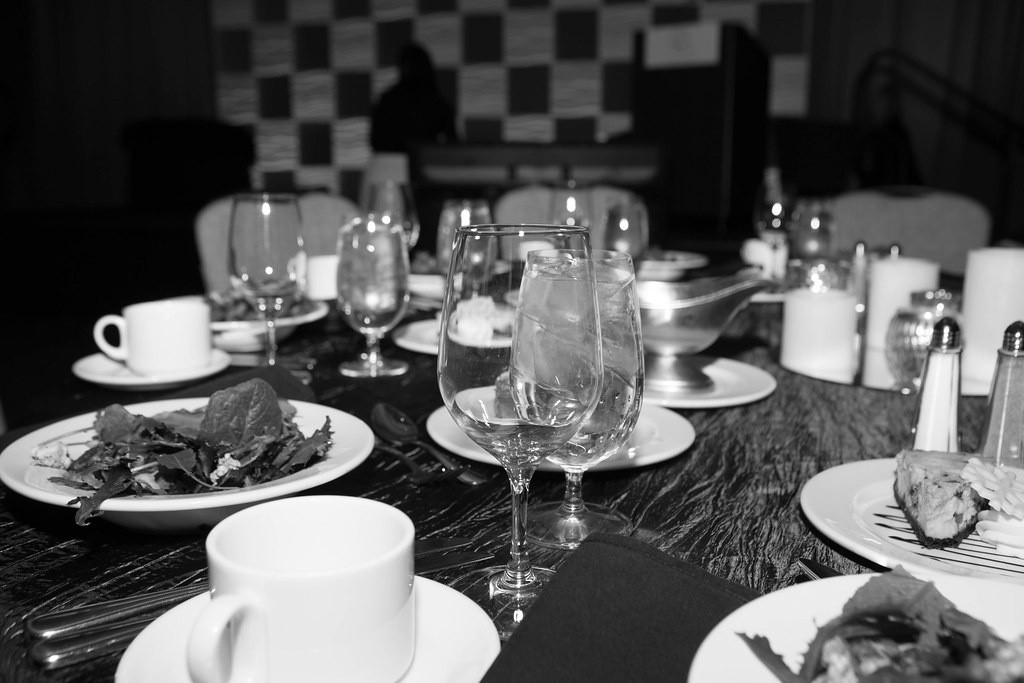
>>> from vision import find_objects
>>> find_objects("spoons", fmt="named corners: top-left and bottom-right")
top-left (370, 401), bottom-right (490, 486)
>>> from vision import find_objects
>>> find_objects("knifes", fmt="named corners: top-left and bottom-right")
top-left (27, 552), bottom-right (494, 670)
top-left (23, 537), bottom-right (471, 642)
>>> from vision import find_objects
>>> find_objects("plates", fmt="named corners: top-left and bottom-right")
top-left (112, 575), bottom-right (501, 682)
top-left (800, 452), bottom-right (1024, 576)
top-left (210, 299), bottom-right (330, 344)
top-left (633, 249), bottom-right (708, 280)
top-left (426, 385), bottom-right (696, 470)
top-left (638, 357), bottom-right (778, 409)
top-left (0, 397), bottom-right (375, 535)
top-left (446, 306), bottom-right (517, 348)
top-left (686, 574), bottom-right (1024, 682)
top-left (391, 316), bottom-right (443, 355)
top-left (71, 349), bottom-right (232, 391)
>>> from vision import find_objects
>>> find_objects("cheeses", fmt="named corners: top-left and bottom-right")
top-left (894, 449), bottom-right (990, 548)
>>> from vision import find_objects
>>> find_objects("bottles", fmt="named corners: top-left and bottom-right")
top-left (905, 317), bottom-right (963, 455)
top-left (978, 320), bottom-right (1024, 465)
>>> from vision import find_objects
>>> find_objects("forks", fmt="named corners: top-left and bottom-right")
top-left (346, 414), bottom-right (473, 483)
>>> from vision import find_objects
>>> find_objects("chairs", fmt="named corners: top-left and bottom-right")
top-left (819, 187), bottom-right (991, 273)
top-left (495, 186), bottom-right (646, 260)
top-left (195, 191), bottom-right (357, 297)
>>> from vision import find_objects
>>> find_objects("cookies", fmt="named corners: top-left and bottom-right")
top-left (960, 457), bottom-right (1024, 560)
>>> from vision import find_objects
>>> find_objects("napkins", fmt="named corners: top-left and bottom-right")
top-left (165, 366), bottom-right (316, 401)
top-left (479, 532), bottom-right (764, 683)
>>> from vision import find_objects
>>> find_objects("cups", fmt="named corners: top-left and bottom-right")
top-left (93, 296), bottom-right (215, 376)
top-left (185, 494), bottom-right (417, 682)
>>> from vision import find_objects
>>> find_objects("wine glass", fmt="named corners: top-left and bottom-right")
top-left (222, 185), bottom-right (847, 643)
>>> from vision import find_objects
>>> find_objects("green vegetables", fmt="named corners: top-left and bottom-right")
top-left (30, 378), bottom-right (335, 526)
top-left (735, 564), bottom-right (1024, 683)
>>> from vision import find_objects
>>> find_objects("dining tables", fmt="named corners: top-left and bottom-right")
top-left (0, 302), bottom-right (991, 683)
